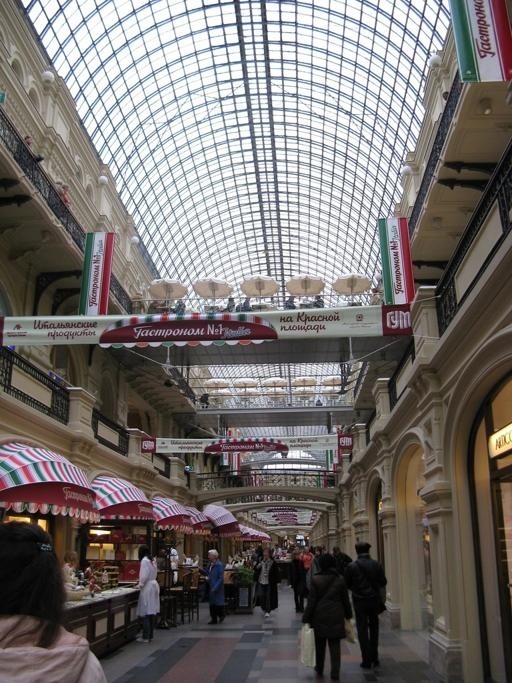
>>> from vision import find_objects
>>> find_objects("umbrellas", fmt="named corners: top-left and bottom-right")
top-left (204, 375), bottom-right (341, 408)
top-left (147, 272), bottom-right (372, 313)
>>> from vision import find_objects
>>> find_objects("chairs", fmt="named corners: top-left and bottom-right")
top-left (156, 568), bottom-right (201, 625)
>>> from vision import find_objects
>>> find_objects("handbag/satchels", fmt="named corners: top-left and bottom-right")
top-left (344, 618), bottom-right (357, 644)
top-left (298, 622), bottom-right (317, 666)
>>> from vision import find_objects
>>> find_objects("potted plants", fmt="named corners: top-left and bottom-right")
top-left (225, 562), bottom-right (257, 615)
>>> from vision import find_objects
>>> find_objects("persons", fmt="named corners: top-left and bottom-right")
top-left (345, 542), bottom-right (388, 669)
top-left (199, 393), bottom-right (210, 409)
top-left (135, 544), bottom-right (161, 644)
top-left (254, 547), bottom-right (280, 618)
top-left (61, 550), bottom-right (80, 582)
top-left (287, 544), bottom-right (351, 680)
top-left (199, 549), bottom-right (226, 625)
top-left (0, 521), bottom-right (107, 683)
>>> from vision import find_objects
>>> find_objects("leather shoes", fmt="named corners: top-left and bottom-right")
top-left (208, 615), bottom-right (225, 624)
top-left (315, 666), bottom-right (324, 675)
top-left (359, 660), bottom-right (379, 670)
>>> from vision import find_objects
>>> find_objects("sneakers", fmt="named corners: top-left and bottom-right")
top-left (136, 632), bottom-right (151, 643)
top-left (264, 611), bottom-right (270, 617)
